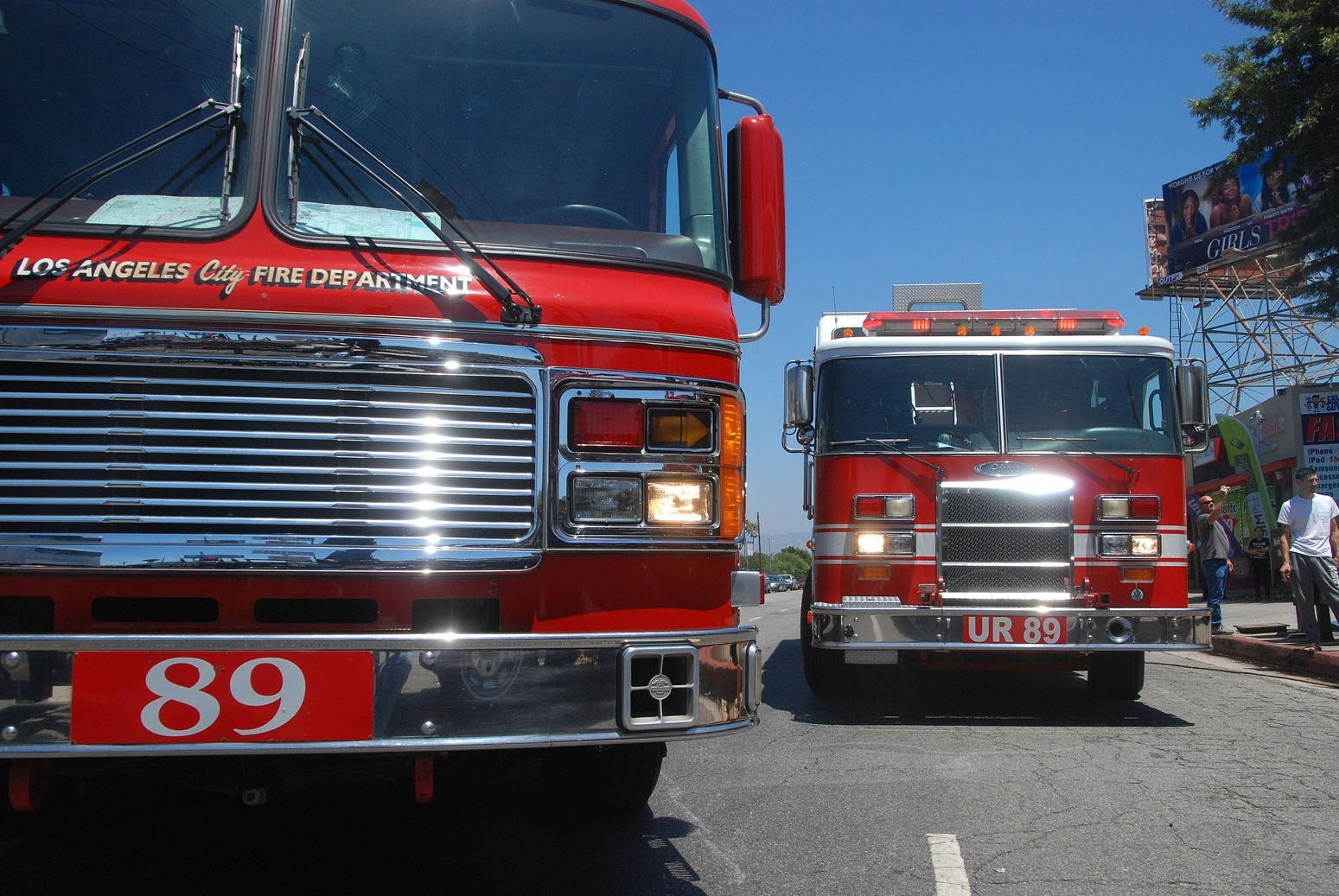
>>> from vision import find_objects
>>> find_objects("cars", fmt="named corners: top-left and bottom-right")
top-left (763, 573), bottom-right (772, 594)
top-left (767, 574), bottom-right (805, 593)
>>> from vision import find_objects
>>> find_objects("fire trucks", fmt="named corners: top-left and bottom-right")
top-left (0, 0), bottom-right (788, 824)
top-left (768, 280), bottom-right (1224, 702)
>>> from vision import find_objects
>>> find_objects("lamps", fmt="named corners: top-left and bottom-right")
top-left (1222, 247), bottom-right (1246, 260)
top-left (1194, 297), bottom-right (1217, 308)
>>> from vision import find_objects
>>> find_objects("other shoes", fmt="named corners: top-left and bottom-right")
top-left (1222, 595), bottom-right (1230, 600)
top-left (1200, 597), bottom-right (1208, 602)
top-left (1255, 598), bottom-right (1260, 602)
top-left (1266, 598), bottom-right (1272, 601)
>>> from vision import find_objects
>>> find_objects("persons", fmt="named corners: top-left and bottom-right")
top-left (1196, 485), bottom-right (1237, 635)
top-left (1248, 527), bottom-right (1273, 604)
top-left (1277, 469), bottom-right (1339, 653)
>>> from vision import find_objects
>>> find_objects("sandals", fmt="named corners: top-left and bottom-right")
top-left (1302, 644), bottom-right (1322, 653)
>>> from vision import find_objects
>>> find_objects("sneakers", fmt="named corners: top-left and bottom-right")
top-left (1211, 626), bottom-right (1234, 635)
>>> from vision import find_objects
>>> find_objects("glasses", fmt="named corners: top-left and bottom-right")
top-left (1204, 499), bottom-right (1214, 504)
